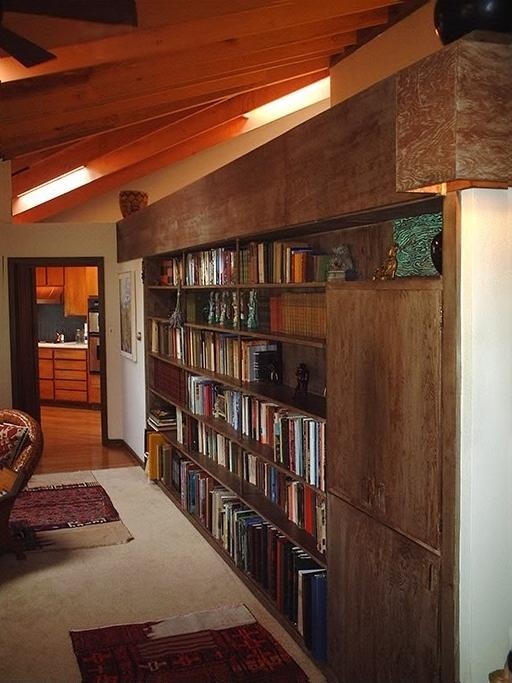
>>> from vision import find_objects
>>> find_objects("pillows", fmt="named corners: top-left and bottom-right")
top-left (0, 422), bottom-right (29, 469)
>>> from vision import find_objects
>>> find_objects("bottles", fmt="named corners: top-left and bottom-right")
top-left (75, 329), bottom-right (82, 344)
top-left (296, 364), bottom-right (311, 396)
top-left (83, 323), bottom-right (88, 344)
top-left (208, 289), bottom-right (260, 329)
top-left (56, 328), bottom-right (64, 343)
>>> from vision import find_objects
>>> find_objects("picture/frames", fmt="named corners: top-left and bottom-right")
top-left (118, 271), bottom-right (138, 361)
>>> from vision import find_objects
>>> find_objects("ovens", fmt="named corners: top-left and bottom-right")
top-left (89, 312), bottom-right (101, 372)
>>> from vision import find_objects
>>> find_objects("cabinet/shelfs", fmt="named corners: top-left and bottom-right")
top-left (64, 267), bottom-right (98, 317)
top-left (35, 267), bottom-right (64, 286)
top-left (38, 348), bottom-right (53, 405)
top-left (86, 348), bottom-right (102, 407)
top-left (141, 32), bottom-right (511, 683)
top-left (53, 348), bottom-right (87, 406)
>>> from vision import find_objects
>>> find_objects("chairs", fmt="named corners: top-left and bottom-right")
top-left (0, 408), bottom-right (44, 560)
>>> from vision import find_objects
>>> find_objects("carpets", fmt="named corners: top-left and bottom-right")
top-left (0, 470), bottom-right (136, 555)
top-left (68, 603), bottom-right (311, 682)
top-left (0, 460), bottom-right (326, 681)
